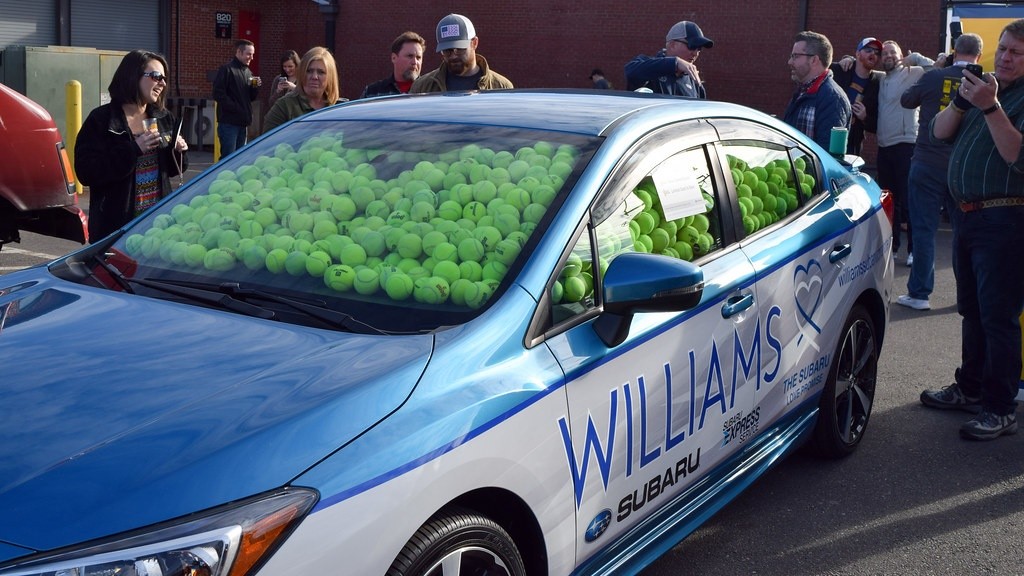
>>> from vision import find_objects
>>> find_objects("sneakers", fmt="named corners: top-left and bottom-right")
top-left (897, 294), bottom-right (930, 310)
top-left (921, 382), bottom-right (983, 413)
top-left (958, 410), bottom-right (1017, 440)
top-left (906, 254), bottom-right (913, 266)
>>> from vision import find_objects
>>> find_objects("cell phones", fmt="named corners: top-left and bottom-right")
top-left (966, 64), bottom-right (982, 85)
top-left (279, 77), bottom-right (287, 81)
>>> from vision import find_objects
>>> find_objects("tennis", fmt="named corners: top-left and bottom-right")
top-left (126, 129), bottom-right (816, 307)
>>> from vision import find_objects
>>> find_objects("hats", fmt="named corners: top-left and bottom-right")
top-left (589, 69), bottom-right (603, 79)
top-left (435, 13), bottom-right (476, 53)
top-left (857, 37), bottom-right (882, 52)
top-left (665, 21), bottom-right (714, 49)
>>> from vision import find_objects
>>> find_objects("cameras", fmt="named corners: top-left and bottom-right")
top-left (944, 16), bottom-right (964, 67)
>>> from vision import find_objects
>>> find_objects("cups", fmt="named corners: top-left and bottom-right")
top-left (830, 127), bottom-right (848, 157)
top-left (143, 118), bottom-right (160, 147)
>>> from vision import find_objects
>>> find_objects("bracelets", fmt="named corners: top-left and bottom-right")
top-left (950, 102), bottom-right (963, 114)
top-left (983, 102), bottom-right (1001, 115)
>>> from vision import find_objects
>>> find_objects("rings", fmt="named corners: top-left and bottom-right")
top-left (965, 89), bottom-right (968, 93)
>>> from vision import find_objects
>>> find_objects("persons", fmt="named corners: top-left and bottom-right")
top-left (588, 70), bottom-right (612, 90)
top-left (831, 36), bottom-right (937, 267)
top-left (359, 31), bottom-right (426, 100)
top-left (623, 20), bottom-right (714, 98)
top-left (767, 30), bottom-right (852, 155)
top-left (898, 33), bottom-right (984, 312)
top-left (409, 12), bottom-right (514, 96)
top-left (920, 20), bottom-right (1024, 441)
top-left (211, 38), bottom-right (263, 162)
top-left (262, 46), bottom-right (348, 134)
top-left (74, 50), bottom-right (190, 242)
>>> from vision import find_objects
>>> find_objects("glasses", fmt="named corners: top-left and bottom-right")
top-left (443, 49), bottom-right (465, 56)
top-left (145, 71), bottom-right (167, 82)
top-left (863, 46), bottom-right (881, 55)
top-left (790, 53), bottom-right (814, 60)
top-left (675, 40), bottom-right (701, 51)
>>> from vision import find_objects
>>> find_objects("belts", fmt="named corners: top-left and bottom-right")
top-left (960, 196), bottom-right (1024, 212)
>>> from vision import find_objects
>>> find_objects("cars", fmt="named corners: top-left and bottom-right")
top-left (0, 86), bottom-right (897, 576)
top-left (0, 80), bottom-right (90, 252)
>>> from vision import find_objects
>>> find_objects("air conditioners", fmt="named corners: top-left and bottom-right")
top-left (185, 106), bottom-right (214, 145)
